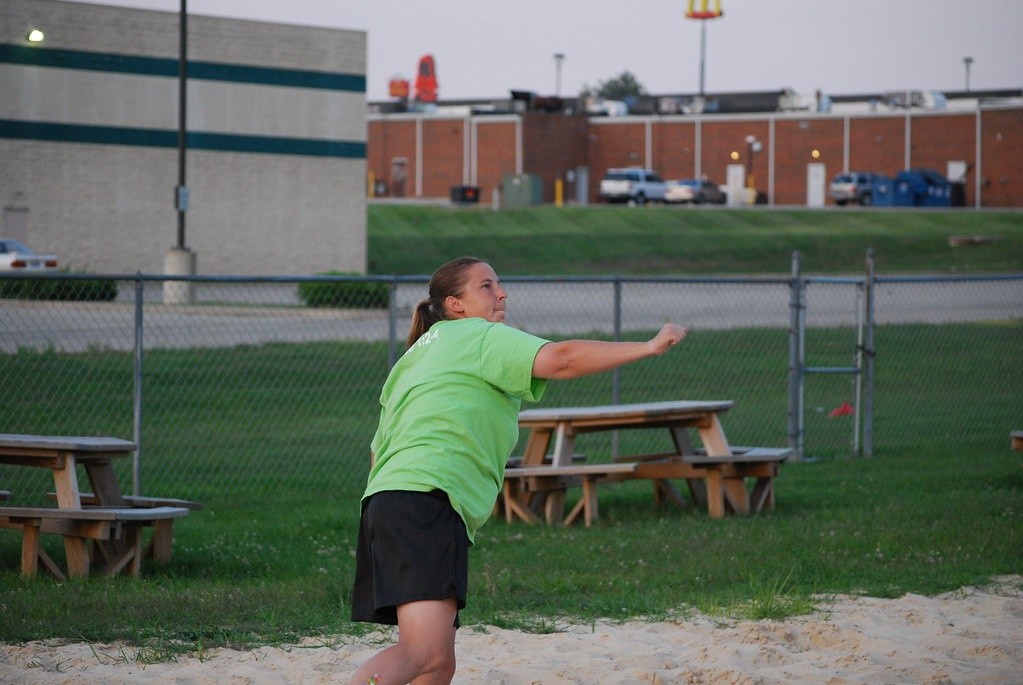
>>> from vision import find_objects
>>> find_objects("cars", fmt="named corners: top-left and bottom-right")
top-left (0, 237), bottom-right (58, 274)
top-left (663, 179), bottom-right (726, 205)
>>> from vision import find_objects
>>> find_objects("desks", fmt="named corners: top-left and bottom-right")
top-left (518, 400), bottom-right (735, 527)
top-left (0, 433), bottom-right (138, 557)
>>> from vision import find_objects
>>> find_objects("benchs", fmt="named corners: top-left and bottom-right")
top-left (491, 446), bottom-right (793, 526)
top-left (0, 491), bottom-right (202, 581)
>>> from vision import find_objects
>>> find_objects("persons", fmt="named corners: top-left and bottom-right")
top-left (344, 257), bottom-right (688, 685)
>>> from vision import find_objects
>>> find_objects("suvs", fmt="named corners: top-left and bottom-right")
top-left (829, 171), bottom-right (888, 205)
top-left (600, 168), bottom-right (666, 206)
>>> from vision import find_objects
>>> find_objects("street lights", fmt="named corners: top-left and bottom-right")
top-left (961, 57), bottom-right (974, 96)
